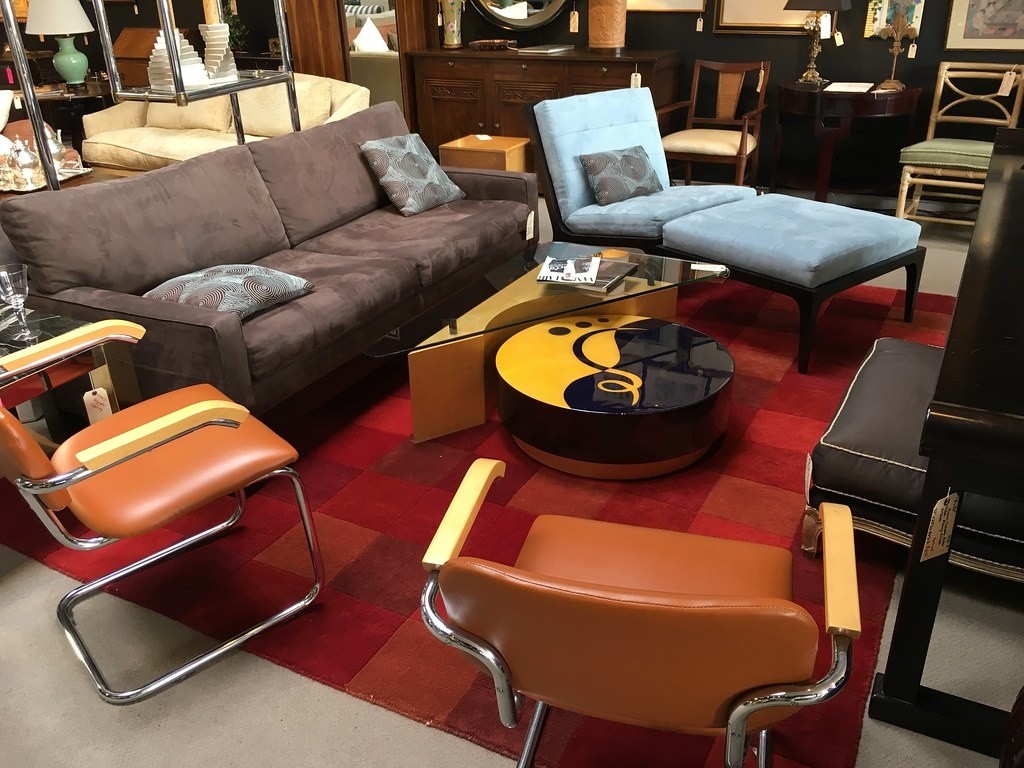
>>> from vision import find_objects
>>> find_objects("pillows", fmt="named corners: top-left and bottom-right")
top-left (228, 78), bottom-right (332, 138)
top-left (352, 18), bottom-right (389, 52)
top-left (388, 31), bottom-right (398, 51)
top-left (0, 90), bottom-right (16, 156)
top-left (579, 146), bottom-right (664, 207)
top-left (357, 133), bottom-right (466, 217)
top-left (145, 94), bottom-right (231, 132)
top-left (141, 264), bottom-right (316, 320)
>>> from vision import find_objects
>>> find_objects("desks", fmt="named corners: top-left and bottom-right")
top-left (14, 81), bottom-right (115, 168)
top-left (769, 84), bottom-right (928, 203)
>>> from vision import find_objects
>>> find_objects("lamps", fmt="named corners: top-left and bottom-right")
top-left (783, 0), bottom-right (852, 86)
top-left (25, 1), bottom-right (95, 92)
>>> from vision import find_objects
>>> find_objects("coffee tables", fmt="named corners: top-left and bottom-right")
top-left (361, 239), bottom-right (736, 480)
top-left (0, 169), bottom-right (128, 199)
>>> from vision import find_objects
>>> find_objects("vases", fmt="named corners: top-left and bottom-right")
top-left (440, 0), bottom-right (463, 48)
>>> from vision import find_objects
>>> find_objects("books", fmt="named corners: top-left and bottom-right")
top-left (518, 44), bottom-right (575, 54)
top-left (537, 255), bottom-right (639, 294)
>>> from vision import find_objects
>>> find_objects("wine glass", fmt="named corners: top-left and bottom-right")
top-left (0, 263), bottom-right (41, 341)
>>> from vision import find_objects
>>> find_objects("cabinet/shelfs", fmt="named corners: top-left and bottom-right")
top-left (405, 44), bottom-right (679, 194)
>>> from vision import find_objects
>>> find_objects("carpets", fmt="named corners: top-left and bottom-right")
top-left (0, 277), bottom-right (957, 768)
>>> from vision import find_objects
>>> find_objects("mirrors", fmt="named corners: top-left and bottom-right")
top-left (471, 0), bottom-right (569, 31)
top-left (343, 1), bottom-right (404, 120)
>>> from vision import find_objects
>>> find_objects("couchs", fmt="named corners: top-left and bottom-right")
top-left (0, 119), bottom-right (82, 167)
top-left (344, 4), bottom-right (404, 114)
top-left (82, 72), bottom-right (370, 172)
top-left (1, 101), bottom-right (539, 499)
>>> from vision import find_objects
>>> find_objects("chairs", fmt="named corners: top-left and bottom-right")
top-left (421, 458), bottom-right (862, 768)
top-left (655, 59), bottom-right (771, 189)
top-left (525, 87), bottom-right (927, 375)
top-left (0, 319), bottom-right (325, 706)
top-left (896, 61), bottom-right (1024, 226)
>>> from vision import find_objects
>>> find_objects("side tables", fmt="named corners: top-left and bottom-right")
top-left (0, 303), bottom-right (143, 411)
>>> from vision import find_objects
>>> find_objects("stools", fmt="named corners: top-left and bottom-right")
top-left (801, 338), bottom-right (1024, 615)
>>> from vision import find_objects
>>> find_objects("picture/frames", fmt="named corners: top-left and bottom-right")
top-left (943, 0), bottom-right (1024, 52)
top-left (712, 0), bottom-right (838, 36)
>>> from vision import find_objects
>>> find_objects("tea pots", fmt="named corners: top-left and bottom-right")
top-left (7, 136), bottom-right (38, 182)
top-left (34, 124), bottom-right (67, 170)
